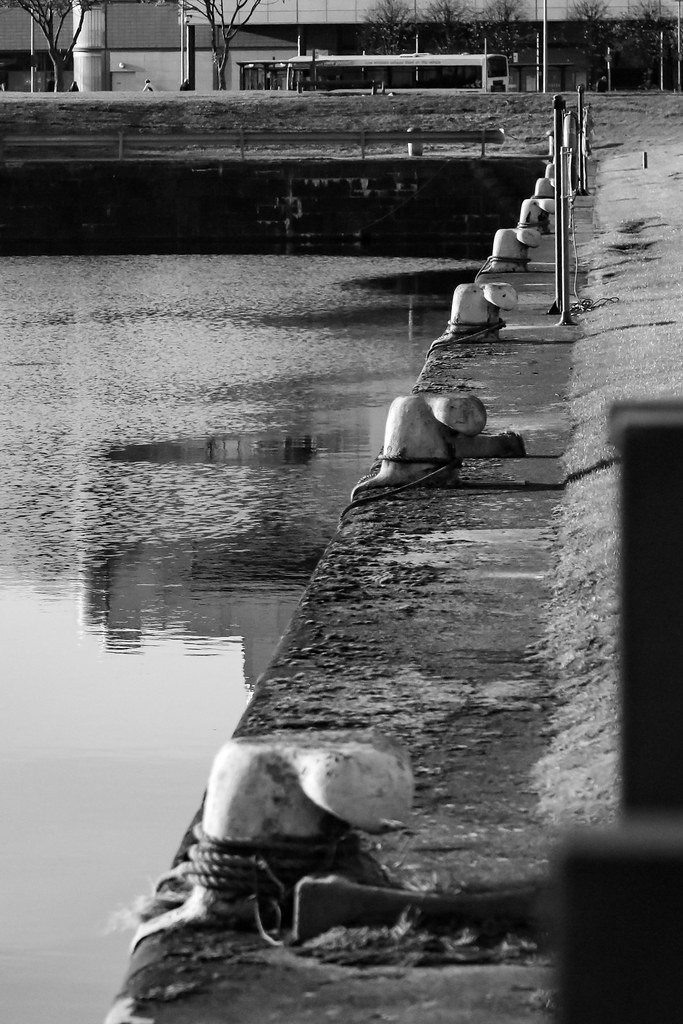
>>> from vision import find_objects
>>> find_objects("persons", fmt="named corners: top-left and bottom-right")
top-left (69, 81), bottom-right (80, 93)
top-left (141, 79), bottom-right (155, 92)
top-left (596, 76), bottom-right (609, 93)
top-left (180, 78), bottom-right (193, 90)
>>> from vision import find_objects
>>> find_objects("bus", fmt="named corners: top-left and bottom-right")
top-left (236, 58), bottom-right (289, 90)
top-left (288, 52), bottom-right (509, 93)
